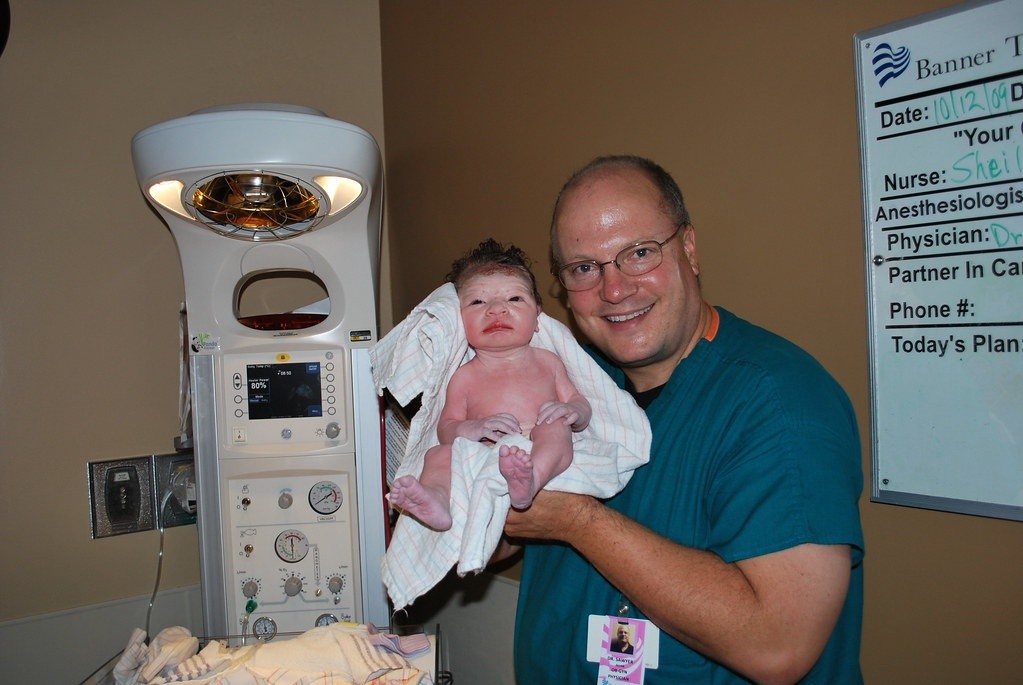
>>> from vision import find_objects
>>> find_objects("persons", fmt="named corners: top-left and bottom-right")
top-left (387, 238), bottom-right (591, 531)
top-left (611, 624), bottom-right (633, 654)
top-left (486, 153), bottom-right (866, 685)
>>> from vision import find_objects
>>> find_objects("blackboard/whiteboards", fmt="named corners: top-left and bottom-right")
top-left (853, 1), bottom-right (1023, 521)
top-left (851, 0), bottom-right (1022, 523)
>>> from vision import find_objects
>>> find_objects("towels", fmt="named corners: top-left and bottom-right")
top-left (367, 282), bottom-right (653, 611)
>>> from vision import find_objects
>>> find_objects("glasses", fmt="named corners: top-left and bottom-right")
top-left (552, 222), bottom-right (686, 292)
